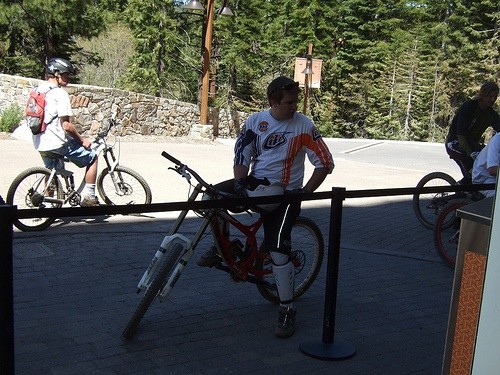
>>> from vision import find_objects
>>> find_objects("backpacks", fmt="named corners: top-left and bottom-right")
top-left (25, 86), bottom-right (61, 135)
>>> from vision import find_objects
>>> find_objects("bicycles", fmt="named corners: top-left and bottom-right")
top-left (5, 112), bottom-right (152, 232)
top-left (434, 200), bottom-right (474, 269)
top-left (412, 172), bottom-right (483, 230)
top-left (122, 151), bottom-right (325, 339)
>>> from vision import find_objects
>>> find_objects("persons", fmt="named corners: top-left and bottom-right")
top-left (196, 77), bottom-right (335, 338)
top-left (33, 58), bottom-right (103, 209)
top-left (445, 82), bottom-right (500, 198)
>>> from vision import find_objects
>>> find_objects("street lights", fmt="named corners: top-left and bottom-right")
top-left (183, 0), bottom-right (234, 143)
top-left (302, 39), bottom-right (315, 115)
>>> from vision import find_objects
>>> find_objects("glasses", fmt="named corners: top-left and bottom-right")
top-left (270, 82), bottom-right (299, 94)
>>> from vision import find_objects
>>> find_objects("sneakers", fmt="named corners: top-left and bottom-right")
top-left (196, 244), bottom-right (223, 267)
top-left (274, 306), bottom-right (298, 338)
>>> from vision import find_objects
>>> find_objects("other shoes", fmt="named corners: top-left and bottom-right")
top-left (80, 196), bottom-right (101, 206)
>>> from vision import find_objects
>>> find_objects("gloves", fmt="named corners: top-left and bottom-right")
top-left (283, 187), bottom-right (309, 199)
top-left (469, 151), bottom-right (479, 160)
top-left (234, 178), bottom-right (246, 196)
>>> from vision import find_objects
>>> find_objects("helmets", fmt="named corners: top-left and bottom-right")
top-left (47, 58), bottom-right (76, 76)
